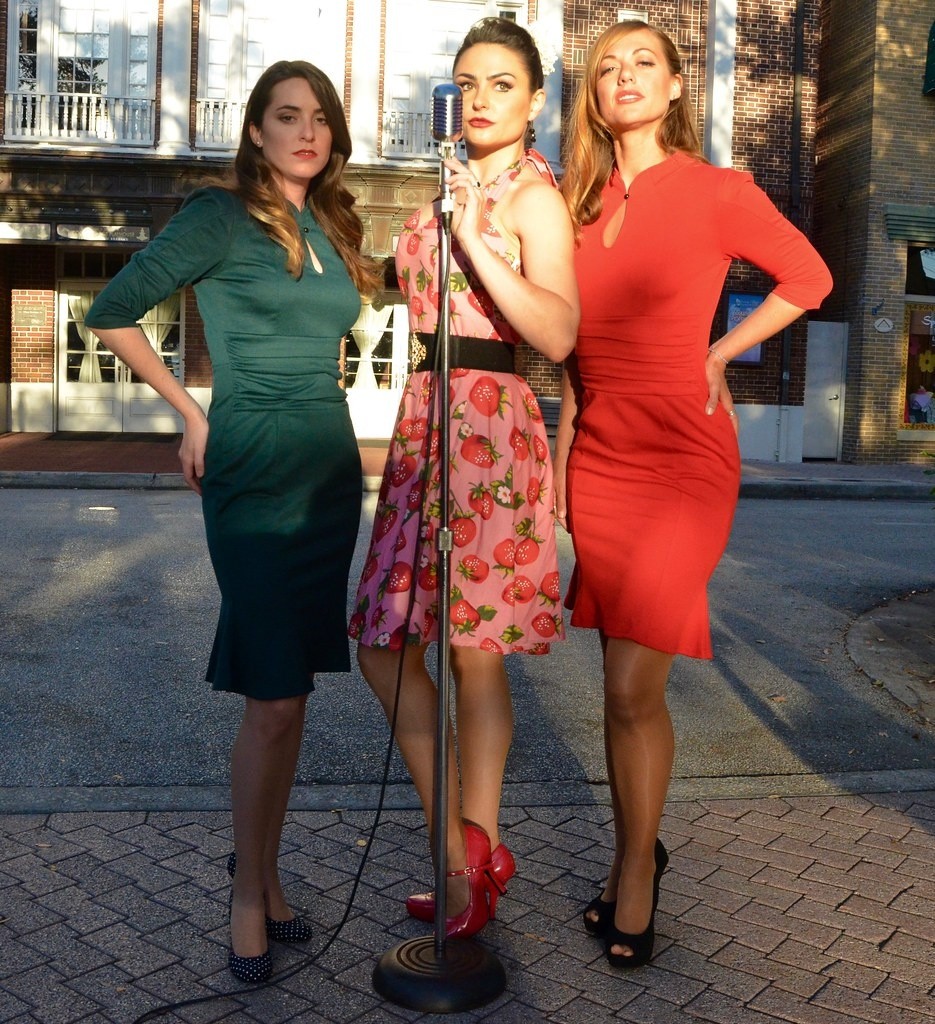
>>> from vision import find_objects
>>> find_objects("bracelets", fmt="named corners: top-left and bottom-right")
top-left (708, 348), bottom-right (731, 366)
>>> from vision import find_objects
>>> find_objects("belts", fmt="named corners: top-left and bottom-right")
top-left (411, 331), bottom-right (515, 373)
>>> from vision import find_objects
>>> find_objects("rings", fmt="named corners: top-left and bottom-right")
top-left (727, 410), bottom-right (737, 417)
top-left (473, 182), bottom-right (483, 190)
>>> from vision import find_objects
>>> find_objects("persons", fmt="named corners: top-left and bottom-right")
top-left (344, 19), bottom-right (576, 948)
top-left (546, 23), bottom-right (837, 974)
top-left (90, 57), bottom-right (386, 986)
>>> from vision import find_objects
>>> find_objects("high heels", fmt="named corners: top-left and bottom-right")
top-left (229, 886), bottom-right (272, 982)
top-left (438, 826), bottom-right (508, 938)
top-left (406, 844), bottom-right (516, 923)
top-left (229, 849), bottom-right (311, 941)
top-left (607, 838), bottom-right (671, 967)
top-left (584, 887), bottom-right (616, 934)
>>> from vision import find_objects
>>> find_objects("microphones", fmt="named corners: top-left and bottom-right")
top-left (431, 84), bottom-right (464, 144)
